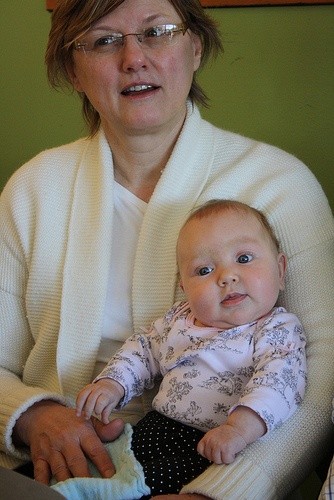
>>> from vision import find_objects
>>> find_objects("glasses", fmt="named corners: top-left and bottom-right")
top-left (66, 22), bottom-right (188, 58)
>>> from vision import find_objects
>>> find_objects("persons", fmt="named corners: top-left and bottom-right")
top-left (0, 0), bottom-right (334, 500)
top-left (74, 197), bottom-right (308, 499)
